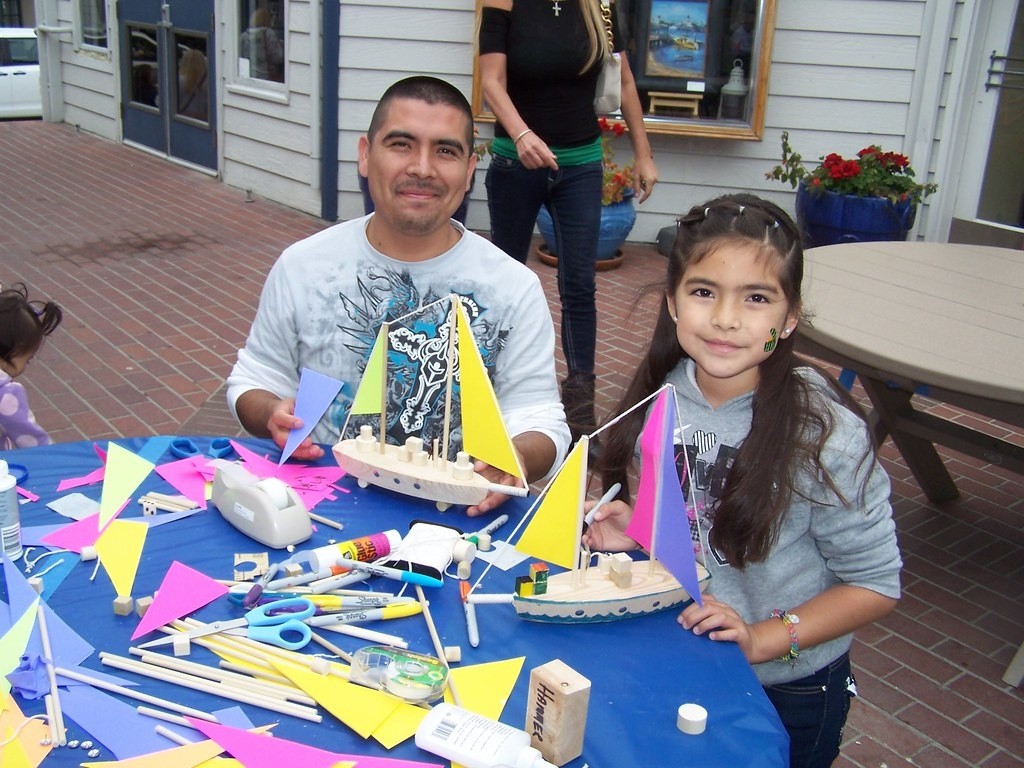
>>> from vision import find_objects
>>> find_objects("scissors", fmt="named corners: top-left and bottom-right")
top-left (136, 597), bottom-right (316, 649)
top-left (170, 437), bottom-right (233, 459)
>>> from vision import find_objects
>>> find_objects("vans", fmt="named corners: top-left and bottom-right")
top-left (0, 27), bottom-right (42, 118)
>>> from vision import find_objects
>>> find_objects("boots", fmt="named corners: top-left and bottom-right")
top-left (562, 371), bottom-right (604, 468)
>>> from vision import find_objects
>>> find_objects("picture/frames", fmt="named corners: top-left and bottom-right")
top-left (632, 0), bottom-right (727, 94)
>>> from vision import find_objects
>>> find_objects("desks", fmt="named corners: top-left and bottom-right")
top-left (790, 238), bottom-right (1024, 508)
top-left (0, 436), bottom-right (792, 768)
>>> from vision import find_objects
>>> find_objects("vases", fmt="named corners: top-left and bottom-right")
top-left (794, 181), bottom-right (917, 250)
top-left (536, 188), bottom-right (637, 271)
top-left (357, 164), bottom-right (476, 226)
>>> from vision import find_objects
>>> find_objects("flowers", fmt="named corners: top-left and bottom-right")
top-left (764, 130), bottom-right (938, 208)
top-left (597, 115), bottom-right (636, 209)
top-left (473, 125), bottom-right (493, 163)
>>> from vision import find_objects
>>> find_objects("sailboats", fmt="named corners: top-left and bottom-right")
top-left (462, 384), bottom-right (713, 625)
top-left (331, 292), bottom-right (530, 506)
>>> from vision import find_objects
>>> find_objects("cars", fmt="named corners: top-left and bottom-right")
top-left (131, 32), bottom-right (159, 69)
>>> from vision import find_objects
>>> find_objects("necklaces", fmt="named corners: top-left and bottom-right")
top-left (551, 0), bottom-right (561, 16)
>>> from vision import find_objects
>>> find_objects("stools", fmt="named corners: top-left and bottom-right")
top-left (647, 92), bottom-right (704, 117)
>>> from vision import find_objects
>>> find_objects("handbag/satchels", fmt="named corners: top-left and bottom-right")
top-left (589, 0), bottom-right (623, 115)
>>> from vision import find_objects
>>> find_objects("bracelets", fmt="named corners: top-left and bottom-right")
top-left (769, 609), bottom-right (799, 663)
top-left (514, 129), bottom-right (531, 146)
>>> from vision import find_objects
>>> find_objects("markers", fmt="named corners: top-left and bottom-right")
top-left (299, 595), bottom-right (416, 606)
top-left (459, 581), bottom-right (479, 647)
top-left (311, 572), bottom-right (378, 594)
top-left (309, 601), bottom-right (424, 626)
top-left (466, 514), bottom-right (509, 545)
top-left (243, 563), bottom-right (279, 608)
top-left (583, 482), bottom-right (622, 537)
top-left (267, 566), bottom-right (349, 590)
top-left (336, 558), bottom-right (444, 588)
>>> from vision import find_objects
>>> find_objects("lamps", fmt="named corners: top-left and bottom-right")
top-left (718, 58), bottom-right (750, 123)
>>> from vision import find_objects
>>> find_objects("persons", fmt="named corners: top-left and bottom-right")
top-left (478, 0), bottom-right (658, 467)
top-left (244, 10), bottom-right (284, 79)
top-left (0, 281), bottom-right (63, 450)
top-left (150, 49), bottom-right (205, 110)
top-left (224, 74), bottom-right (572, 516)
top-left (579, 192), bottom-right (902, 768)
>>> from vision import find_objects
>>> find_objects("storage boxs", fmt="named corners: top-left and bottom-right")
top-left (523, 658), bottom-right (591, 767)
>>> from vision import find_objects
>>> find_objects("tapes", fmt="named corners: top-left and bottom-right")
top-left (205, 458), bottom-right (289, 509)
top-left (385, 680), bottom-right (433, 700)
